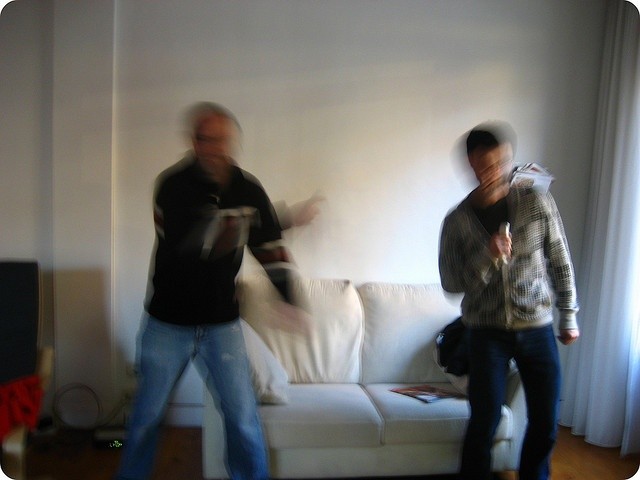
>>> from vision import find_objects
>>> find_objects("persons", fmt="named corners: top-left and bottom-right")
top-left (114, 103), bottom-right (326, 480)
top-left (438, 120), bottom-right (581, 478)
